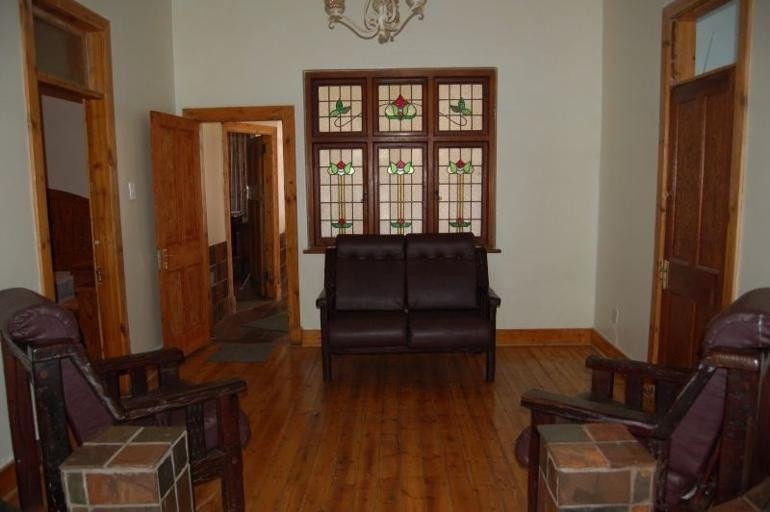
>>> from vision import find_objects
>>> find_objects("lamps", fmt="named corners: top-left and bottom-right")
top-left (324, 0), bottom-right (427, 45)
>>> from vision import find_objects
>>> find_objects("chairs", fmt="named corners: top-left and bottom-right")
top-left (0, 287), bottom-right (251, 512)
top-left (514, 287), bottom-right (770, 512)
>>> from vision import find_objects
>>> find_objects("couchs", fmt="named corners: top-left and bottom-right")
top-left (315, 232), bottom-right (501, 382)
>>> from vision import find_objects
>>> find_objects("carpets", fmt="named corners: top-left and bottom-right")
top-left (204, 344), bottom-right (275, 363)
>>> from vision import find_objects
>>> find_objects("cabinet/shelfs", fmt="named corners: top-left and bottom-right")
top-left (58, 425), bottom-right (195, 512)
top-left (536, 423), bottom-right (658, 512)
